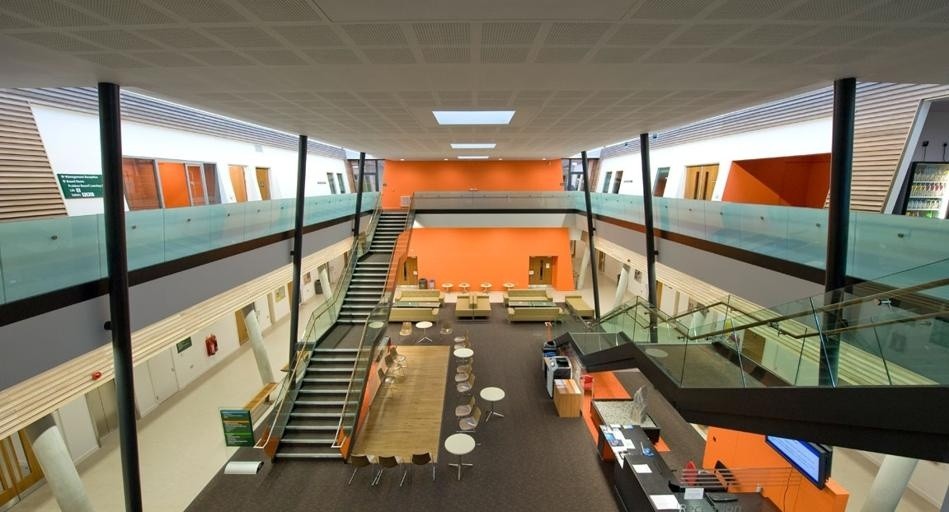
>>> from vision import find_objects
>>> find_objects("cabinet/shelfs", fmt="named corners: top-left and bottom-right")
top-left (553, 379), bottom-right (581, 418)
top-left (901, 161), bottom-right (949, 218)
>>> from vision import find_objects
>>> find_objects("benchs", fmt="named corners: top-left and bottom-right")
top-left (565, 296), bottom-right (594, 319)
top-left (244, 382), bottom-right (280, 415)
top-left (280, 351), bottom-right (309, 372)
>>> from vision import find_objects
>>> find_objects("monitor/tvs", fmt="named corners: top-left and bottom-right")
top-left (764, 435), bottom-right (833, 491)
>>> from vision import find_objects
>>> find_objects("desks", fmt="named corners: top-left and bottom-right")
top-left (479, 386), bottom-right (506, 423)
top-left (597, 424), bottom-right (782, 512)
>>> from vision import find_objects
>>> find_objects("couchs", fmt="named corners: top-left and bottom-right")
top-left (389, 289), bottom-right (563, 326)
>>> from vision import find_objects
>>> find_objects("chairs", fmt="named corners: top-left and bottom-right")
top-left (348, 320), bottom-right (482, 487)
top-left (579, 375), bottom-right (594, 397)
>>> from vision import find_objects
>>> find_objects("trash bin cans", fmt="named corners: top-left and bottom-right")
top-left (541, 340), bottom-right (557, 371)
top-left (419, 278), bottom-right (427, 289)
